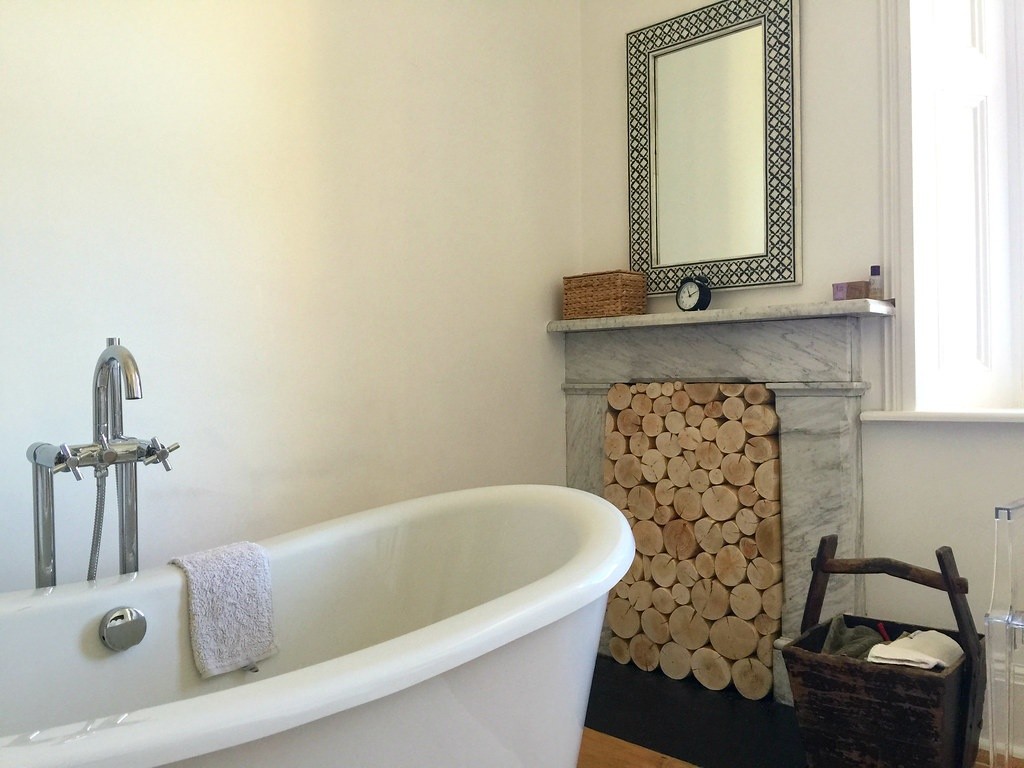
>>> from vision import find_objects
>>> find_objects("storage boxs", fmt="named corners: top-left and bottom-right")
top-left (560, 270), bottom-right (648, 320)
top-left (781, 535), bottom-right (988, 768)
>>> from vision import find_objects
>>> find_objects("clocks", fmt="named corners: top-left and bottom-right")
top-left (675, 275), bottom-right (712, 311)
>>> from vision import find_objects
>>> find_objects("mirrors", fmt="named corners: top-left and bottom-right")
top-left (653, 22), bottom-right (768, 267)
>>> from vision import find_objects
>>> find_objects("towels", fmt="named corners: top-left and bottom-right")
top-left (166, 539), bottom-right (282, 681)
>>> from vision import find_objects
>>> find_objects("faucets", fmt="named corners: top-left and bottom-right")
top-left (92, 344), bottom-right (144, 447)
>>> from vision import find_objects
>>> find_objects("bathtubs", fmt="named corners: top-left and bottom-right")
top-left (0, 483), bottom-right (636, 768)
